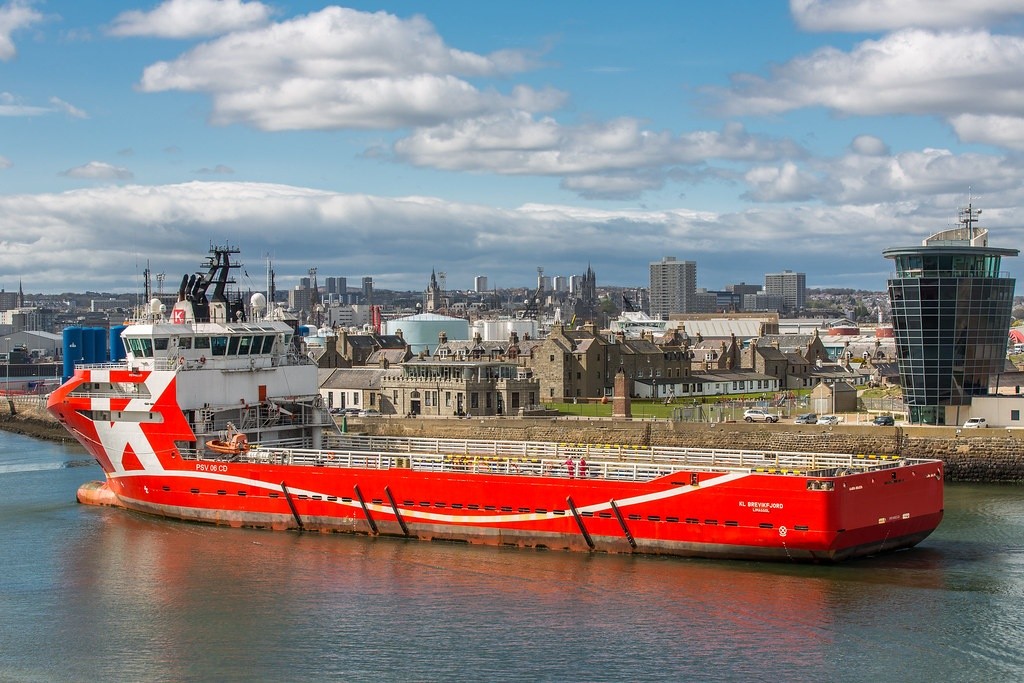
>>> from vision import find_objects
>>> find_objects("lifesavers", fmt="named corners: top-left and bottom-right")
top-left (179, 355), bottom-right (187, 365)
top-left (327, 452), bottom-right (334, 461)
top-left (199, 355), bottom-right (207, 364)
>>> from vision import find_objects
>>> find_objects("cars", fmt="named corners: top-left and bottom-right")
top-left (818, 415), bottom-right (838, 426)
top-left (963, 417), bottom-right (989, 428)
top-left (1008, 344), bottom-right (1023, 355)
top-left (872, 416), bottom-right (895, 426)
top-left (330, 407), bottom-right (360, 417)
top-left (794, 414), bottom-right (818, 424)
top-left (743, 410), bottom-right (779, 423)
top-left (358, 409), bottom-right (383, 418)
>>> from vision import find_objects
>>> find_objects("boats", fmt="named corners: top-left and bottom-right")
top-left (48, 372), bottom-right (945, 563)
top-left (205, 422), bottom-right (250, 455)
top-left (81, 244), bottom-right (321, 410)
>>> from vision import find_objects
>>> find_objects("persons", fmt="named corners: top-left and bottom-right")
top-left (561, 456), bottom-right (575, 479)
top-left (579, 456), bottom-right (586, 479)
top-left (198, 355), bottom-right (205, 364)
top-left (406, 411), bottom-right (416, 418)
top-left (694, 397), bottom-right (696, 402)
top-left (180, 357), bottom-right (184, 365)
top-left (702, 397), bottom-right (705, 403)
top-left (459, 411), bottom-right (465, 418)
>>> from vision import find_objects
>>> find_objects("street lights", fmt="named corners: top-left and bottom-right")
top-left (796, 378), bottom-right (800, 396)
